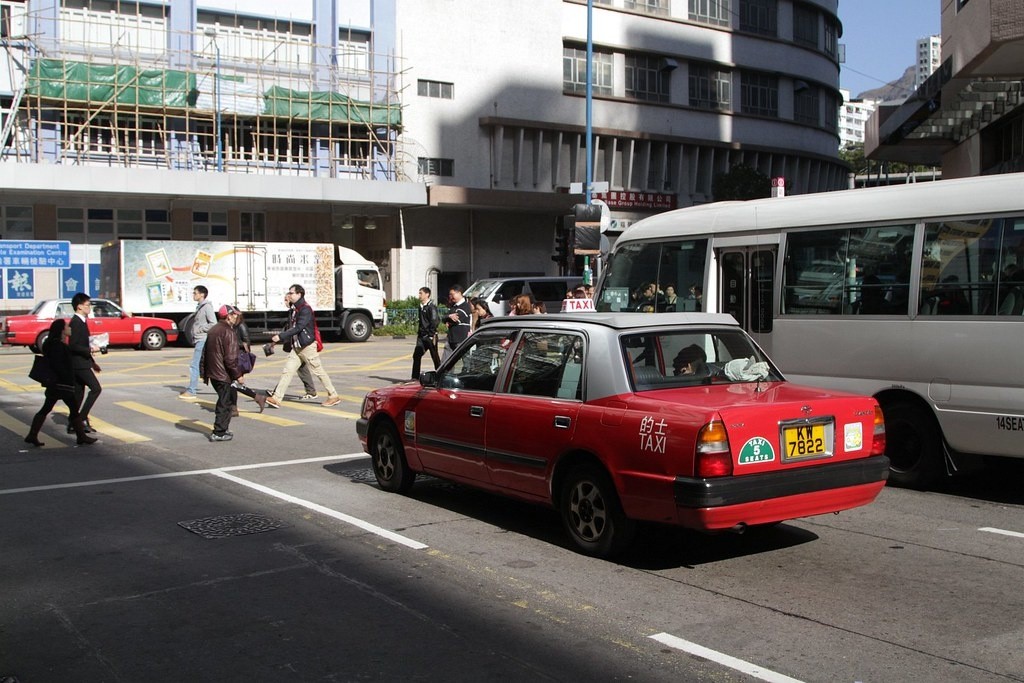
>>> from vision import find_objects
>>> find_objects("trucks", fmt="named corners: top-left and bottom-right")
top-left (100, 239), bottom-right (387, 342)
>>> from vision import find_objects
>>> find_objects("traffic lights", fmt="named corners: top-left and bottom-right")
top-left (551, 236), bottom-right (567, 266)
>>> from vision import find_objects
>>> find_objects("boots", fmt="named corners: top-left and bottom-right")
top-left (25, 415), bottom-right (46, 447)
top-left (69, 413), bottom-right (97, 444)
top-left (255, 393), bottom-right (266, 413)
top-left (231, 405), bottom-right (238, 417)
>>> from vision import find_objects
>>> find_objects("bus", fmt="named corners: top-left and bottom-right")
top-left (591, 172), bottom-right (1024, 488)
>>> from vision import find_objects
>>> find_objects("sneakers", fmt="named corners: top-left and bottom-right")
top-left (265, 397), bottom-right (280, 408)
top-left (322, 397), bottom-right (340, 406)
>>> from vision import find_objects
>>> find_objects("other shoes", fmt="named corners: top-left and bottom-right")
top-left (209, 433), bottom-right (232, 442)
top-left (299, 394), bottom-right (317, 399)
top-left (68, 424), bottom-right (90, 434)
top-left (82, 419), bottom-right (96, 433)
top-left (267, 389), bottom-right (274, 396)
top-left (179, 392), bottom-right (196, 399)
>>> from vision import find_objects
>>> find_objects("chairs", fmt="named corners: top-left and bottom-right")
top-left (634, 365), bottom-right (668, 390)
top-left (696, 361), bottom-right (730, 381)
top-left (920, 302), bottom-right (931, 315)
top-left (998, 293), bottom-right (1015, 315)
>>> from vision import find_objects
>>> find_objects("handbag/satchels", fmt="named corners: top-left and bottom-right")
top-left (28, 350), bottom-right (48, 388)
top-left (314, 330), bottom-right (323, 352)
top-left (238, 349), bottom-right (256, 373)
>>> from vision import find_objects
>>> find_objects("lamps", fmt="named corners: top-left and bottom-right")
top-left (341, 216), bottom-right (354, 229)
top-left (364, 216), bottom-right (377, 230)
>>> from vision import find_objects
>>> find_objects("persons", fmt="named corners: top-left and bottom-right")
top-left (23, 318), bottom-right (98, 446)
top-left (504, 296), bottom-right (551, 360)
top-left (199, 305), bottom-right (244, 441)
top-left (627, 277), bottom-right (702, 309)
top-left (410, 287), bottom-right (441, 380)
top-left (94, 307), bottom-right (105, 317)
top-left (440, 285), bottom-right (500, 375)
top-left (65, 291), bottom-right (104, 434)
top-left (920, 262), bottom-right (1024, 317)
top-left (179, 285), bottom-right (218, 398)
top-left (564, 284), bottom-right (597, 300)
top-left (263, 284), bottom-right (342, 409)
top-left (228, 306), bottom-right (267, 416)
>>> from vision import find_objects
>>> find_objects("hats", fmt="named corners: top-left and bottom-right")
top-left (218, 305), bottom-right (242, 316)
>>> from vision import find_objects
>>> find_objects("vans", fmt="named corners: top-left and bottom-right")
top-left (455, 277), bottom-right (599, 317)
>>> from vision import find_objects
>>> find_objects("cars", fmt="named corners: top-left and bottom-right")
top-left (0, 298), bottom-right (178, 354)
top-left (357, 298), bottom-right (890, 558)
